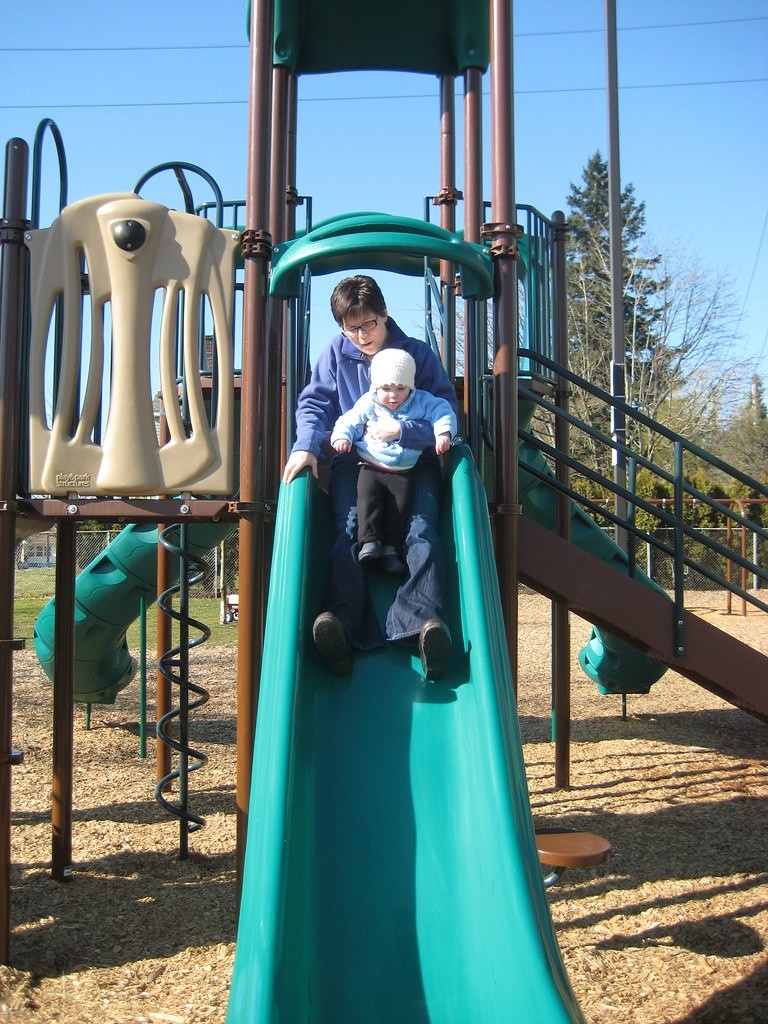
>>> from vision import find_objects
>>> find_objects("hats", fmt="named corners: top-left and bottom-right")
top-left (371, 348), bottom-right (416, 390)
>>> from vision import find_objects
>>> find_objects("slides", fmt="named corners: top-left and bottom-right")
top-left (512, 398), bottom-right (676, 694)
top-left (222, 441), bottom-right (586, 1024)
top-left (33, 514), bottom-right (238, 705)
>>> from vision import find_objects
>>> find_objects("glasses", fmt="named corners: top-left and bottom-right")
top-left (341, 312), bottom-right (380, 337)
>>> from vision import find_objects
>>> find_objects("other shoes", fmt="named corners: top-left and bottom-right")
top-left (378, 554), bottom-right (405, 573)
top-left (358, 540), bottom-right (382, 561)
top-left (313, 611), bottom-right (353, 675)
top-left (419, 618), bottom-right (451, 681)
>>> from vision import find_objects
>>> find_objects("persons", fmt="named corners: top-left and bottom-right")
top-left (282, 271), bottom-right (459, 681)
top-left (329, 347), bottom-right (456, 575)
